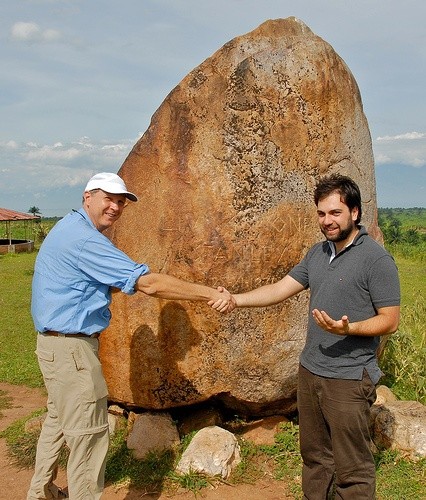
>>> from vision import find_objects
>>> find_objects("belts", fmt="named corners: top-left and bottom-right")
top-left (39, 330), bottom-right (101, 339)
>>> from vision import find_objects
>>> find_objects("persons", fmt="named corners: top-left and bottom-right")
top-left (207, 172), bottom-right (401, 500)
top-left (25, 172), bottom-right (236, 500)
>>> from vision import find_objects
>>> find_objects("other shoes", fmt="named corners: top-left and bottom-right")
top-left (50, 487), bottom-right (68, 500)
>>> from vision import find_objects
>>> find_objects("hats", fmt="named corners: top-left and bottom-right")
top-left (84, 172), bottom-right (138, 202)
